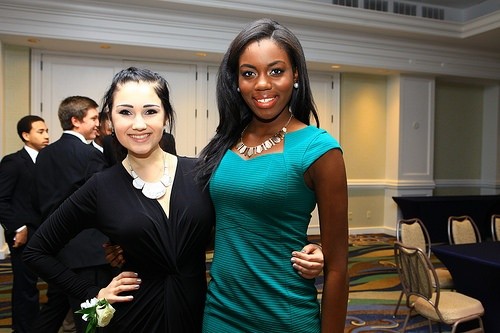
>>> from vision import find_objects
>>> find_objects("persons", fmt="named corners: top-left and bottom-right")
top-left (89, 113), bottom-right (177, 167)
top-left (0, 96), bottom-right (107, 333)
top-left (21, 66), bottom-right (325, 333)
top-left (103, 17), bottom-right (350, 333)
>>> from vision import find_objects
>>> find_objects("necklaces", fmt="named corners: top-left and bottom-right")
top-left (127, 154), bottom-right (172, 199)
top-left (235, 111), bottom-right (293, 157)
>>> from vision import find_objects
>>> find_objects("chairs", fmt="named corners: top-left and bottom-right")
top-left (393, 218), bottom-right (453, 318)
top-left (491, 214), bottom-right (500, 243)
top-left (447, 214), bottom-right (481, 245)
top-left (394, 241), bottom-right (485, 333)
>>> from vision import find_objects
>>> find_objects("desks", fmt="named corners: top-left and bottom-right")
top-left (391, 194), bottom-right (500, 243)
top-left (431, 242), bottom-right (500, 333)
